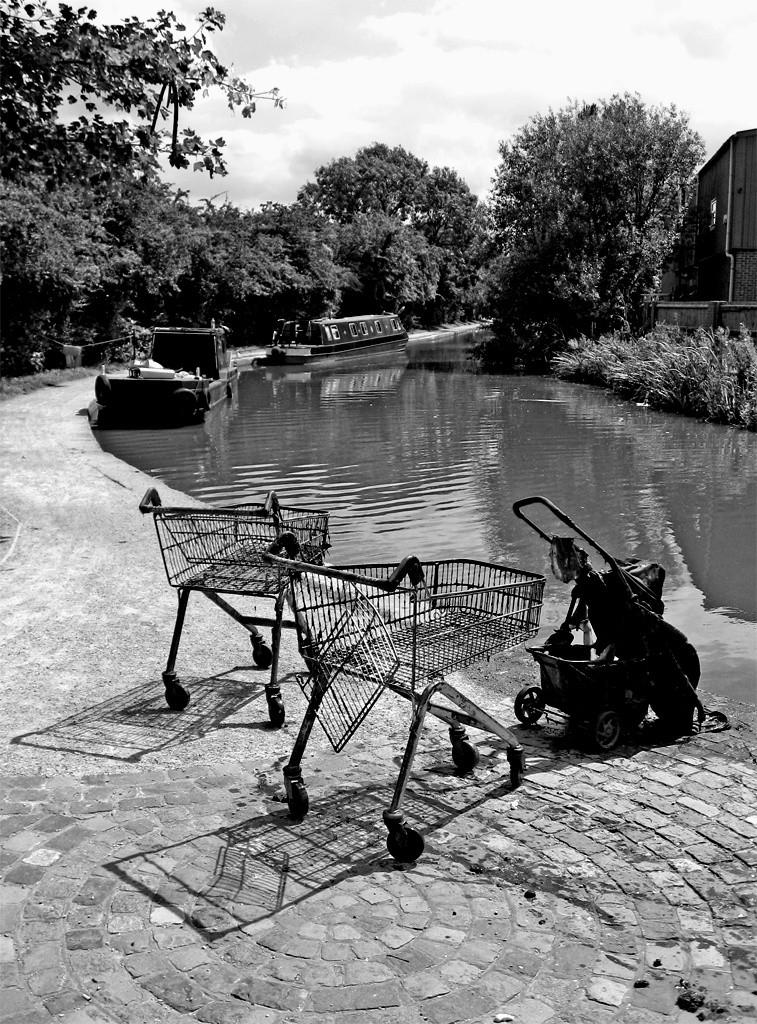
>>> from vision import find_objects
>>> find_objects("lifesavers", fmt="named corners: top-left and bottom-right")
top-left (94, 374), bottom-right (112, 406)
top-left (200, 387), bottom-right (212, 411)
top-left (169, 388), bottom-right (199, 417)
top-left (227, 382), bottom-right (233, 399)
top-left (271, 347), bottom-right (288, 361)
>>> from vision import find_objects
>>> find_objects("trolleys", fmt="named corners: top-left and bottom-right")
top-left (257, 530), bottom-right (549, 865)
top-left (139, 488), bottom-right (329, 729)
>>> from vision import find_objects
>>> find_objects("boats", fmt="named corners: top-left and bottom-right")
top-left (248, 312), bottom-right (409, 368)
top-left (93, 318), bottom-right (242, 424)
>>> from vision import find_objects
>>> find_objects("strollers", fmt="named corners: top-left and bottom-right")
top-left (512, 496), bottom-right (732, 750)
